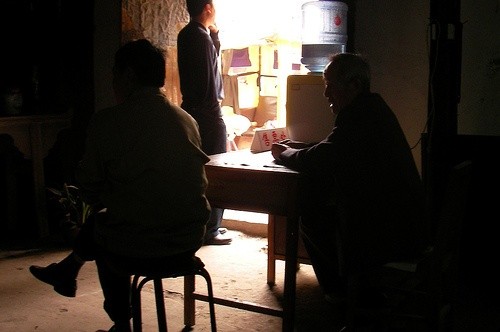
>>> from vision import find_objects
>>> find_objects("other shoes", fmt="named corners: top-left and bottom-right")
top-left (206, 227), bottom-right (234, 246)
top-left (28, 262), bottom-right (77, 298)
top-left (93, 322), bottom-right (133, 331)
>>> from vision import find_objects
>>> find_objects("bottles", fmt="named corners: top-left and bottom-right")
top-left (301, 1), bottom-right (348, 73)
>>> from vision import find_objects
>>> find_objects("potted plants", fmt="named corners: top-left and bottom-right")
top-left (47, 186), bottom-right (93, 245)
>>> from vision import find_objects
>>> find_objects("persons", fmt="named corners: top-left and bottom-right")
top-left (176, 0), bottom-right (233, 245)
top-left (29, 38), bottom-right (210, 332)
top-left (271, 53), bottom-right (425, 332)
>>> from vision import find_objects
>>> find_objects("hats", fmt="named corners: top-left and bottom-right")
top-left (325, 51), bottom-right (372, 91)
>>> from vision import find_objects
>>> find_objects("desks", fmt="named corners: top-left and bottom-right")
top-left (199, 137), bottom-right (317, 315)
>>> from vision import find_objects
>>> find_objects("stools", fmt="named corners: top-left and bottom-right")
top-left (126, 256), bottom-right (217, 332)
